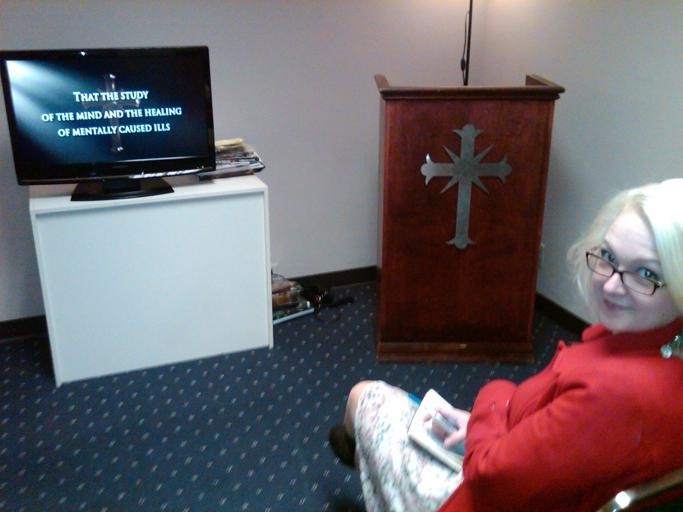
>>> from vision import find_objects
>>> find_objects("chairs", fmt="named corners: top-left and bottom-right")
top-left (597, 470), bottom-right (683, 512)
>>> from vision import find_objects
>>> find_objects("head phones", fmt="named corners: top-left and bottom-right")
top-left (302, 277), bottom-right (330, 306)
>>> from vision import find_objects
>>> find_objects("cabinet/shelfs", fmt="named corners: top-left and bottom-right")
top-left (29, 176), bottom-right (274, 390)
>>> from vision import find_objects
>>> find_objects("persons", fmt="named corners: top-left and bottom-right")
top-left (329, 178), bottom-right (683, 512)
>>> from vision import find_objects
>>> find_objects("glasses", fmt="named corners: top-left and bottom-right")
top-left (585, 242), bottom-right (667, 296)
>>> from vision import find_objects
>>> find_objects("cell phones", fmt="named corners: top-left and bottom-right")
top-left (430, 412), bottom-right (466, 455)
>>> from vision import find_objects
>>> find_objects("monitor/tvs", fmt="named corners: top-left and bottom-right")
top-left (0, 45), bottom-right (214, 201)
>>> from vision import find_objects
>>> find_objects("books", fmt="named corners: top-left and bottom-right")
top-left (194, 137), bottom-right (267, 182)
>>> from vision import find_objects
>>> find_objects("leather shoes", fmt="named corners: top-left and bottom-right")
top-left (327, 423), bottom-right (355, 470)
